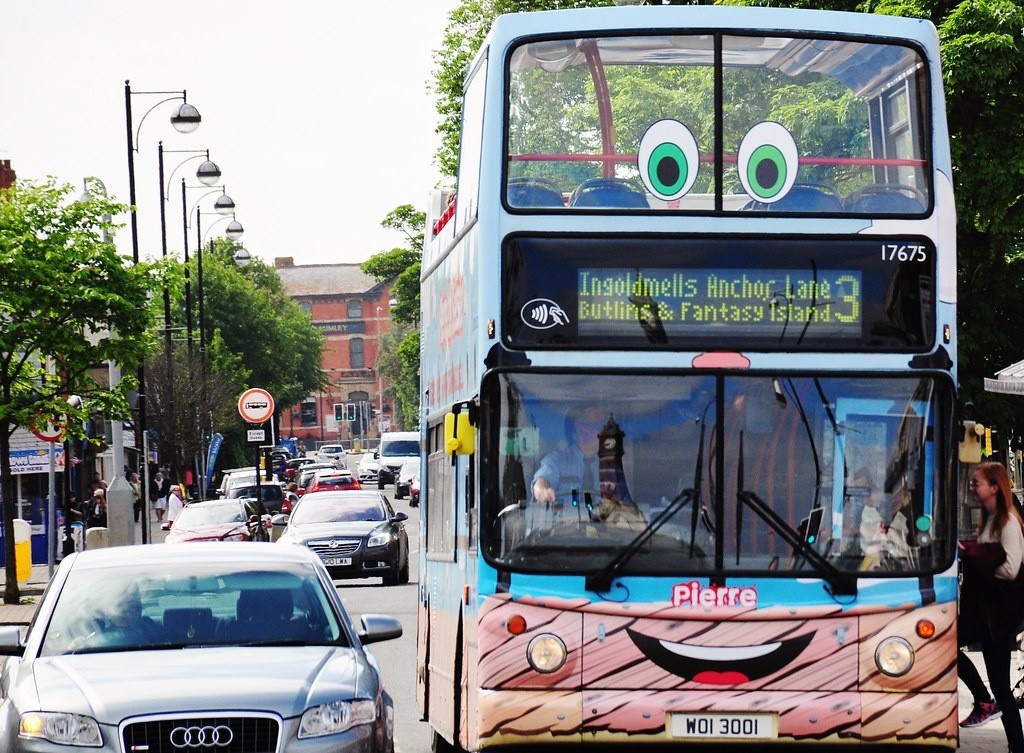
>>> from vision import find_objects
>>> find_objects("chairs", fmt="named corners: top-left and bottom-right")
top-left (507, 177), bottom-right (650, 208)
top-left (737, 182), bottom-right (927, 213)
top-left (236, 589), bottom-right (293, 627)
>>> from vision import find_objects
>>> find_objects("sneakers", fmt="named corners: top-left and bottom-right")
top-left (959, 698), bottom-right (1002, 728)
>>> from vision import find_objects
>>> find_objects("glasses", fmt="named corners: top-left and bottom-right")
top-left (968, 480), bottom-right (986, 489)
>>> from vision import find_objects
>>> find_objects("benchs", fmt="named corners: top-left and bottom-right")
top-left (141, 606), bottom-right (235, 642)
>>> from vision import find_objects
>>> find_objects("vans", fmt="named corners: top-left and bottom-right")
top-left (373, 432), bottom-right (421, 490)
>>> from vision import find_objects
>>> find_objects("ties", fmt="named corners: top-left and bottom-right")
top-left (582, 456), bottom-right (595, 491)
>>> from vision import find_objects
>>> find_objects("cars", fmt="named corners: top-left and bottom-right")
top-left (392, 461), bottom-right (421, 500)
top-left (160, 494), bottom-right (273, 541)
top-left (270, 489), bottom-right (410, 586)
top-left (214, 444), bottom-right (364, 517)
top-left (355, 451), bottom-right (381, 484)
top-left (408, 467), bottom-right (421, 508)
top-left (0, 541), bottom-right (406, 753)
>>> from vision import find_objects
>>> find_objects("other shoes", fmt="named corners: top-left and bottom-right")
top-left (157, 518), bottom-right (162, 523)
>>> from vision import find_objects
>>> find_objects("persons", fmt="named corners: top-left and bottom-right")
top-left (167, 485), bottom-right (187, 527)
top-left (85, 471), bottom-right (109, 528)
top-left (61, 492), bottom-right (84, 542)
top-left (129, 473), bottom-right (142, 522)
top-left (957, 462), bottom-right (1024, 753)
top-left (299, 441), bottom-right (307, 458)
top-left (527, 404), bottom-right (614, 503)
top-left (124, 465), bottom-right (135, 482)
top-left (149, 472), bottom-right (170, 523)
top-left (286, 483), bottom-right (300, 508)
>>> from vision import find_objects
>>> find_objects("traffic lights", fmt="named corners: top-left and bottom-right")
top-left (346, 403), bottom-right (355, 421)
top-left (334, 404), bottom-right (343, 421)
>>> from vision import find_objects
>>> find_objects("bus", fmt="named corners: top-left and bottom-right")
top-left (413, 3), bottom-right (993, 753)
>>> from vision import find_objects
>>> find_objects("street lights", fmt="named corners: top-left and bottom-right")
top-left (181, 177), bottom-right (236, 413)
top-left (159, 139), bottom-right (224, 488)
top-left (123, 77), bottom-right (205, 539)
top-left (195, 203), bottom-right (246, 444)
